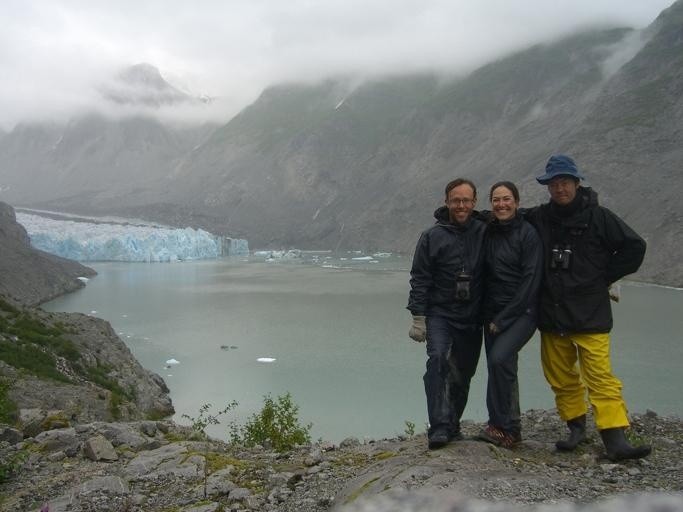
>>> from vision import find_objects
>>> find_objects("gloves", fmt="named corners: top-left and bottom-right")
top-left (489, 322), bottom-right (497, 335)
top-left (408, 316), bottom-right (427, 343)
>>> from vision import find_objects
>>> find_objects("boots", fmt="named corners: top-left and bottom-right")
top-left (555, 414), bottom-right (587, 450)
top-left (600, 428), bottom-right (651, 462)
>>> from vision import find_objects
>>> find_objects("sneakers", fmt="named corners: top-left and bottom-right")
top-left (479, 426), bottom-right (522, 448)
top-left (428, 424), bottom-right (459, 448)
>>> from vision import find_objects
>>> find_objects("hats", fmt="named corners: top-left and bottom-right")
top-left (536, 155), bottom-right (583, 185)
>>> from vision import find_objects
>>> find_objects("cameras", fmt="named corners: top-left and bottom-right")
top-left (550, 243), bottom-right (577, 270)
top-left (454, 272), bottom-right (472, 304)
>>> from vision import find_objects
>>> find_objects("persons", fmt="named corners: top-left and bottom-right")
top-left (406, 178), bottom-right (487, 449)
top-left (435, 156), bottom-right (652, 463)
top-left (470, 182), bottom-right (544, 448)
